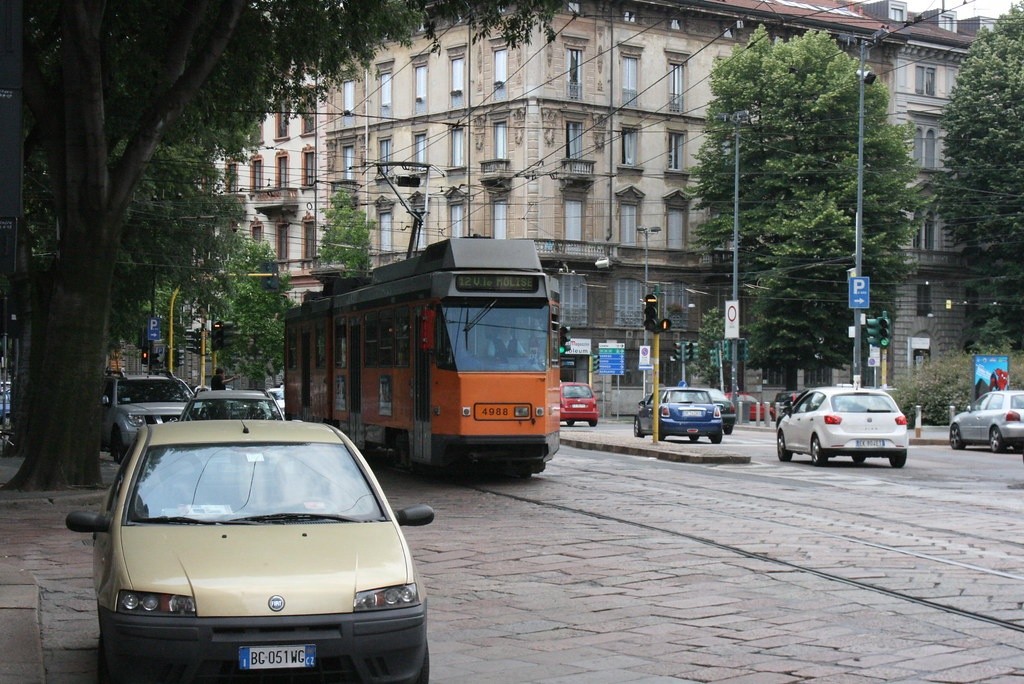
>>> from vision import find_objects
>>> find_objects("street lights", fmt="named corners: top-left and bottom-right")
top-left (867, 318), bottom-right (879, 347)
top-left (635, 225), bottom-right (661, 392)
top-left (716, 109), bottom-right (752, 400)
top-left (878, 318), bottom-right (891, 348)
top-left (837, 27), bottom-right (891, 386)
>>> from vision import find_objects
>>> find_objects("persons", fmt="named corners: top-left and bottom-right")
top-left (489, 318), bottom-right (527, 358)
top-left (211, 366), bottom-right (241, 390)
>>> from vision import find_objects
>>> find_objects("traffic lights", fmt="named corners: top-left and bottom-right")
top-left (141, 348), bottom-right (148, 365)
top-left (176, 348), bottom-right (184, 367)
top-left (643, 294), bottom-right (658, 331)
top-left (220, 320), bottom-right (234, 347)
top-left (186, 328), bottom-right (199, 353)
top-left (658, 319), bottom-right (672, 333)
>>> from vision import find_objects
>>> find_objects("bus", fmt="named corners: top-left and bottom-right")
top-left (277, 237), bottom-right (562, 482)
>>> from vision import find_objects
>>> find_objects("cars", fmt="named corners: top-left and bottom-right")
top-left (949, 390), bottom-right (1024, 453)
top-left (705, 388), bottom-right (737, 435)
top-left (178, 385), bottom-right (285, 423)
top-left (65, 419), bottom-right (437, 684)
top-left (724, 392), bottom-right (776, 421)
top-left (560, 382), bottom-right (600, 427)
top-left (633, 388), bottom-right (724, 443)
top-left (776, 387), bottom-right (909, 468)
top-left (775, 392), bottom-right (803, 421)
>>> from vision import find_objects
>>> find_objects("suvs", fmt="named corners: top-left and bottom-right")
top-left (101, 369), bottom-right (194, 464)
